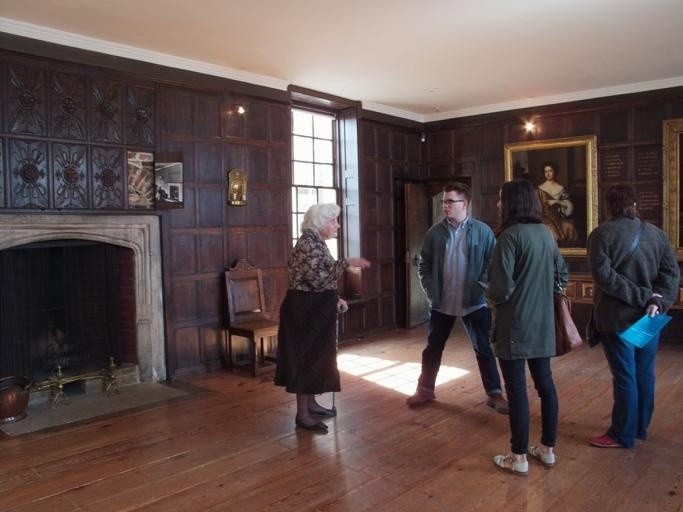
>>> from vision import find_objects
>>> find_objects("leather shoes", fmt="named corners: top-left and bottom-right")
top-left (295, 416), bottom-right (328, 430)
top-left (492, 455), bottom-right (528, 476)
top-left (554, 284), bottom-right (582, 357)
top-left (527, 446), bottom-right (555, 467)
top-left (587, 435), bottom-right (622, 448)
top-left (308, 408), bottom-right (337, 416)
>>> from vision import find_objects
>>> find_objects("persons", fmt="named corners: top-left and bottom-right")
top-left (405, 181), bottom-right (510, 415)
top-left (587, 184), bottom-right (680, 449)
top-left (484, 178), bottom-right (570, 477)
top-left (275, 203), bottom-right (370, 430)
top-left (536, 162), bottom-right (578, 242)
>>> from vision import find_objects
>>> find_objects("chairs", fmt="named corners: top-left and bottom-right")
top-left (223, 259), bottom-right (280, 377)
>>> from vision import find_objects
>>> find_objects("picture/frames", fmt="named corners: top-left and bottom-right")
top-left (503, 134), bottom-right (599, 259)
top-left (661, 119), bottom-right (682, 263)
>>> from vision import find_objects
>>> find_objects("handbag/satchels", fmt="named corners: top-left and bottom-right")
top-left (585, 308), bottom-right (599, 349)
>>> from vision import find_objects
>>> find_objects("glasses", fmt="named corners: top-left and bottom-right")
top-left (438, 199), bottom-right (463, 207)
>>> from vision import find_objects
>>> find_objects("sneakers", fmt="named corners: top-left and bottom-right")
top-left (486, 395), bottom-right (509, 413)
top-left (406, 393), bottom-right (435, 405)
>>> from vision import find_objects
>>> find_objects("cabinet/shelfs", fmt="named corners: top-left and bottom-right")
top-left (564, 274), bottom-right (595, 305)
